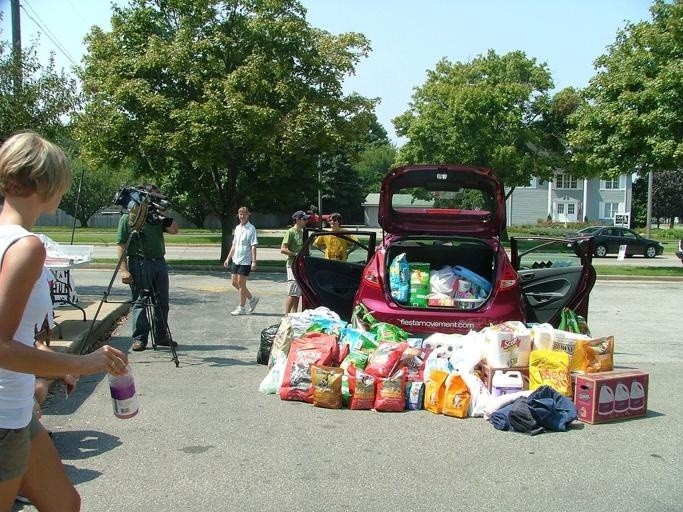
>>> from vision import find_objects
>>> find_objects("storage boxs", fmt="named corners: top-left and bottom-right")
top-left (574, 369), bottom-right (649, 424)
top-left (481, 363), bottom-right (529, 394)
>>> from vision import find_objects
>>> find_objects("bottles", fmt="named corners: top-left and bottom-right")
top-left (107, 358), bottom-right (138, 420)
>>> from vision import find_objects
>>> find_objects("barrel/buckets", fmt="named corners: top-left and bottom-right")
top-left (629, 379), bottom-right (646, 411)
top-left (491, 369), bottom-right (524, 398)
top-left (597, 382), bottom-right (614, 415)
top-left (614, 381), bottom-right (630, 413)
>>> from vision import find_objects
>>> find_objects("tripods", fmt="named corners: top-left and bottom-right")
top-left (78, 229), bottom-right (181, 367)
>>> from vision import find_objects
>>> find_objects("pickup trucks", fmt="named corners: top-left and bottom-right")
top-left (309, 209), bottom-right (330, 227)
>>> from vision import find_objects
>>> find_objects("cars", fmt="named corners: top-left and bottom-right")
top-left (290, 164), bottom-right (596, 338)
top-left (570, 226), bottom-right (664, 257)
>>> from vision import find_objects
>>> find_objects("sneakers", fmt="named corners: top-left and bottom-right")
top-left (130, 338), bottom-right (146, 351)
top-left (229, 306), bottom-right (246, 315)
top-left (153, 334), bottom-right (177, 346)
top-left (247, 294), bottom-right (259, 313)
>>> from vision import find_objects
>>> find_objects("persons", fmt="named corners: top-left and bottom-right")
top-left (280, 210), bottom-right (311, 320)
top-left (116, 185), bottom-right (178, 351)
top-left (33, 339), bottom-right (75, 437)
top-left (223, 206), bottom-right (259, 315)
top-left (312, 212), bottom-right (361, 262)
top-left (0, 131), bottom-right (128, 512)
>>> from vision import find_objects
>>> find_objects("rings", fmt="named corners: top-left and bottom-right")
top-left (110, 361), bottom-right (115, 368)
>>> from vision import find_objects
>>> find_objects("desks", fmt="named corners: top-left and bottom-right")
top-left (44, 258), bottom-right (93, 322)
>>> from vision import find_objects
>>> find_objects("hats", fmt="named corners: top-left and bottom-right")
top-left (291, 211), bottom-right (310, 221)
